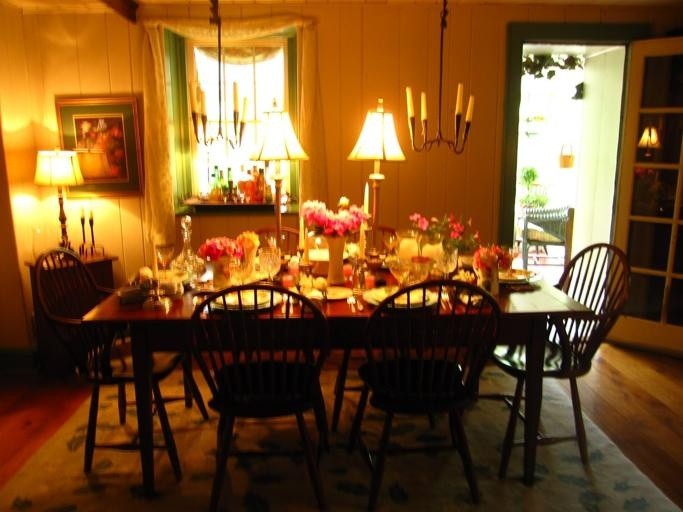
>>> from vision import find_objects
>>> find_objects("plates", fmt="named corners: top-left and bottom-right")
top-left (499, 267), bottom-right (536, 283)
top-left (210, 290), bottom-right (282, 311)
top-left (361, 286), bottom-right (439, 310)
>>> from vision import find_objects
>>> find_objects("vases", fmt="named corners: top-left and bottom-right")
top-left (323, 234), bottom-right (345, 285)
top-left (209, 253), bottom-right (232, 290)
top-left (476, 266), bottom-right (501, 294)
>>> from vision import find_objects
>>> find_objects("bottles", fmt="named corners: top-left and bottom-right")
top-left (206, 164), bottom-right (272, 203)
top-left (168, 216), bottom-right (206, 278)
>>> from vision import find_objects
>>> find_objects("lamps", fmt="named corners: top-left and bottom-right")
top-left (398, 1), bottom-right (476, 159)
top-left (245, 95), bottom-right (314, 243)
top-left (637, 118), bottom-right (663, 165)
top-left (347, 96), bottom-right (408, 231)
top-left (30, 142), bottom-right (85, 254)
top-left (183, 0), bottom-right (255, 151)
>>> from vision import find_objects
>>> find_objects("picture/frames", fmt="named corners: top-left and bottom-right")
top-left (51, 90), bottom-right (144, 201)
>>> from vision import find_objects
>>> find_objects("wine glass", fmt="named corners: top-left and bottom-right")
top-left (504, 240), bottom-right (521, 279)
top-left (154, 230), bottom-right (458, 299)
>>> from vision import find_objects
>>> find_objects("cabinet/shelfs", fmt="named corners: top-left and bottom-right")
top-left (23, 252), bottom-right (122, 374)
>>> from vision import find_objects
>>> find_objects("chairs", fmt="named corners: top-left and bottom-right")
top-left (332, 225), bottom-right (403, 433)
top-left (344, 275), bottom-right (507, 511)
top-left (30, 242), bottom-right (210, 481)
top-left (211, 222), bottom-right (329, 445)
top-left (469, 241), bottom-right (635, 487)
top-left (516, 201), bottom-right (575, 279)
top-left (182, 281), bottom-right (337, 510)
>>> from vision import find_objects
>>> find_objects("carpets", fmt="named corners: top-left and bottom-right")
top-left (0, 368), bottom-right (681, 511)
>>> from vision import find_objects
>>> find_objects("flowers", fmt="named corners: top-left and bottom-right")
top-left (194, 234), bottom-right (241, 259)
top-left (470, 240), bottom-right (513, 276)
top-left (299, 197), bottom-right (367, 237)
top-left (409, 206), bottom-right (480, 248)
